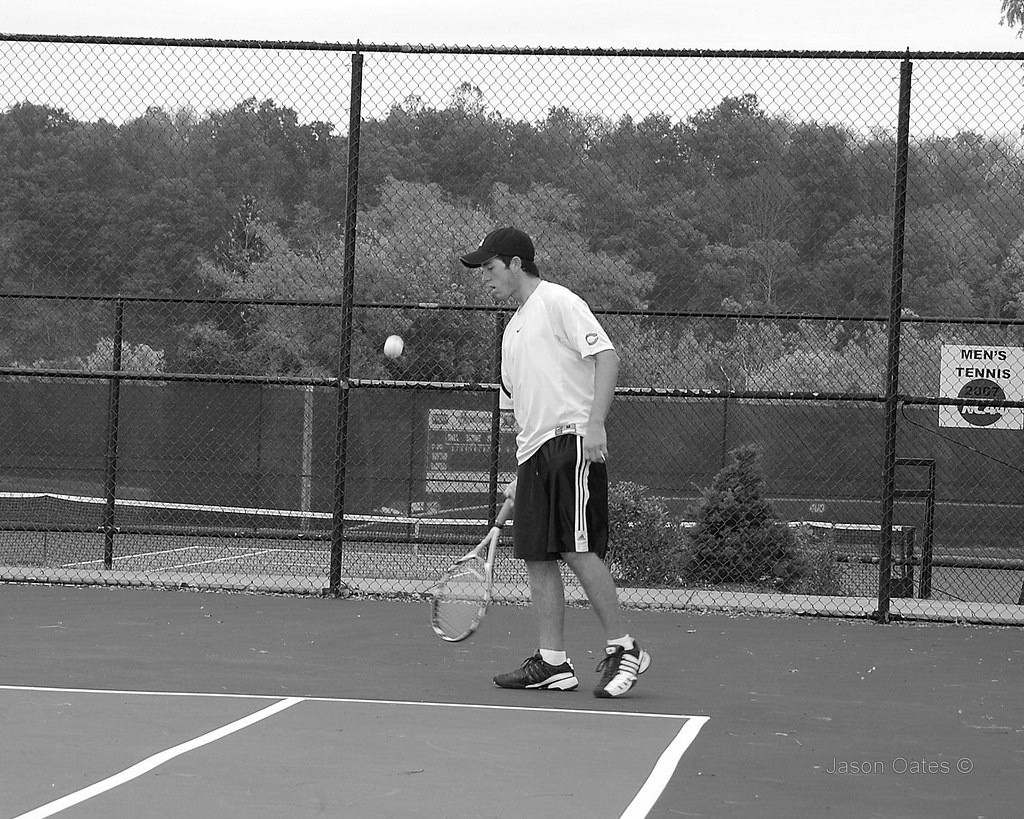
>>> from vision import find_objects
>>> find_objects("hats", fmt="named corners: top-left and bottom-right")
top-left (460, 226), bottom-right (534, 269)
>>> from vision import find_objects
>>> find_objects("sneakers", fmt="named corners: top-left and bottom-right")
top-left (493, 648), bottom-right (579, 691)
top-left (593, 640), bottom-right (651, 698)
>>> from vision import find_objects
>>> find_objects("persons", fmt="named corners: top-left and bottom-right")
top-left (459, 225), bottom-right (651, 698)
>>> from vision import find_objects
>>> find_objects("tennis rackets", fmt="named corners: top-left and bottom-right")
top-left (430, 496), bottom-right (516, 646)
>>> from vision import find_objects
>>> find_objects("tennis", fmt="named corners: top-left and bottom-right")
top-left (381, 333), bottom-right (405, 361)
